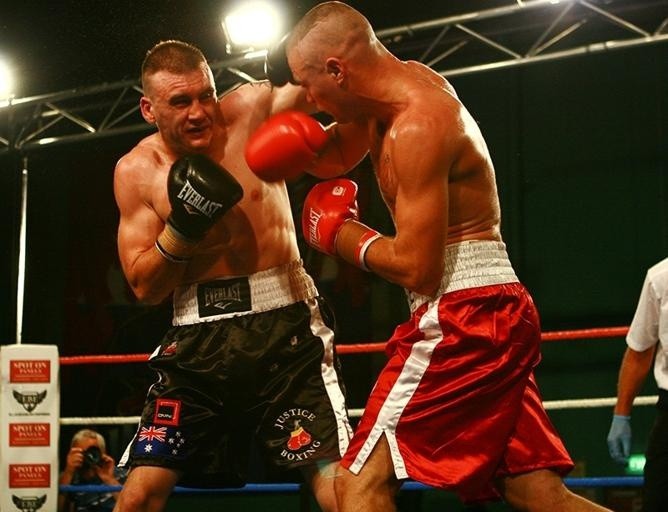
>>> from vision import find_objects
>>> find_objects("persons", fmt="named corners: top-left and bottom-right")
top-left (608, 258), bottom-right (667, 507)
top-left (113, 40), bottom-right (355, 512)
top-left (249, 2), bottom-right (611, 512)
top-left (56, 430), bottom-right (123, 507)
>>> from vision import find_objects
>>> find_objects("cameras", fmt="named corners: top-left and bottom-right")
top-left (80, 445), bottom-right (104, 468)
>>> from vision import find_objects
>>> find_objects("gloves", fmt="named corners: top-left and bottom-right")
top-left (242, 112), bottom-right (329, 183)
top-left (167, 153), bottom-right (244, 243)
top-left (302, 177), bottom-right (383, 273)
top-left (264, 32), bottom-right (301, 88)
top-left (607, 415), bottom-right (632, 461)
